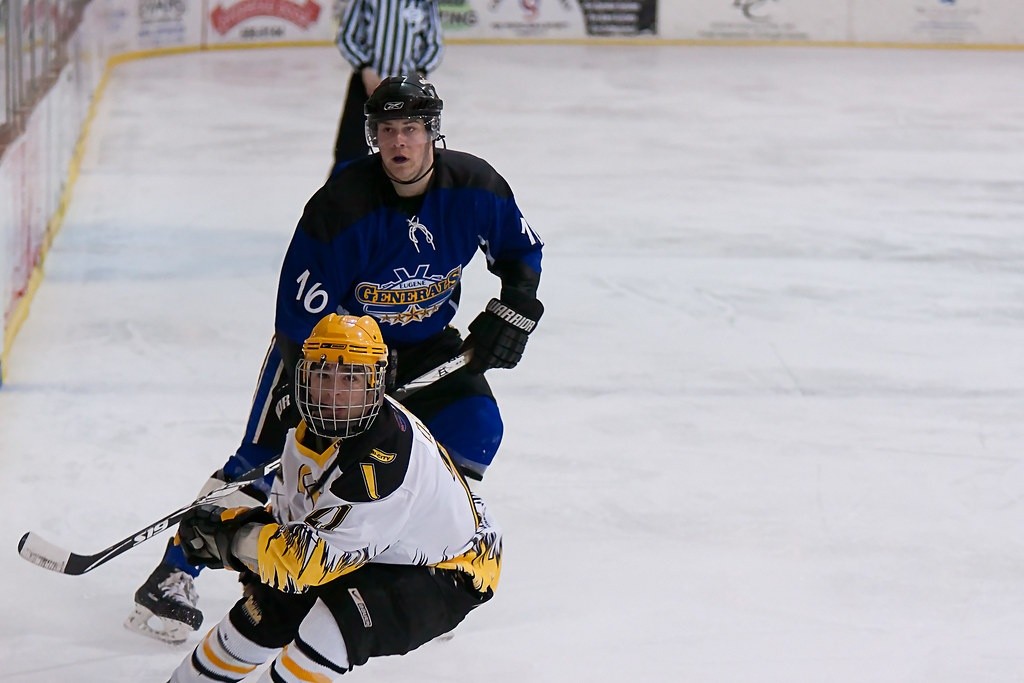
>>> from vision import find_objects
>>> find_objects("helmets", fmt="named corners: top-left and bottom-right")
top-left (364, 74), bottom-right (444, 146)
top-left (299, 312), bottom-right (389, 438)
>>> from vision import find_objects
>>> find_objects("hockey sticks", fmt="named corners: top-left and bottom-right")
top-left (15, 350), bottom-right (477, 577)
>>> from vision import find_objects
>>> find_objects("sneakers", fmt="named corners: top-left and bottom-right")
top-left (124, 563), bottom-right (204, 644)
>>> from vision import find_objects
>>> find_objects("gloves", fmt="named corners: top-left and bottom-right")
top-left (455, 292), bottom-right (544, 376)
top-left (172, 503), bottom-right (281, 573)
top-left (270, 379), bottom-right (301, 429)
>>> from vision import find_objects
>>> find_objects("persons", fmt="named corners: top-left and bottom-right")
top-left (331, 2), bottom-right (441, 161)
top-left (131, 76), bottom-right (543, 643)
top-left (161, 315), bottom-right (501, 683)
